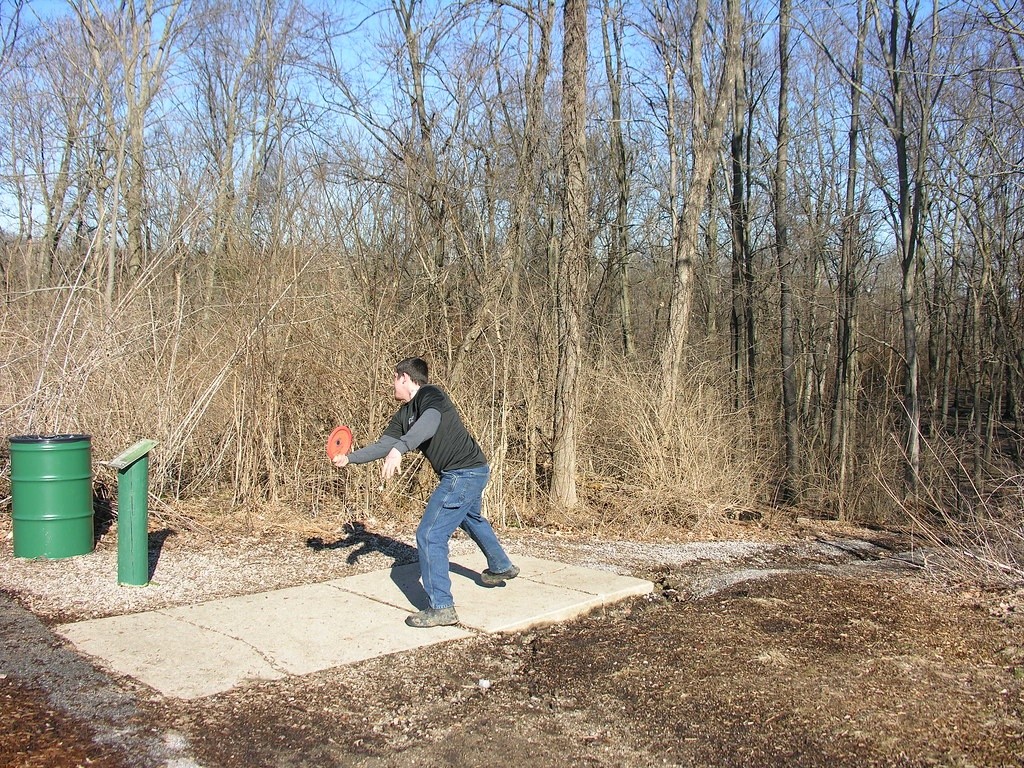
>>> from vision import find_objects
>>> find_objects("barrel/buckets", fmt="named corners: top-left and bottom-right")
top-left (8, 435), bottom-right (94, 558)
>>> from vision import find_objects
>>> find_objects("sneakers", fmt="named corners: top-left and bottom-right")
top-left (481, 565), bottom-right (520, 585)
top-left (405, 606), bottom-right (459, 627)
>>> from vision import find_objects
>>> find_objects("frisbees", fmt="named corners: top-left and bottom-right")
top-left (327, 426), bottom-right (353, 460)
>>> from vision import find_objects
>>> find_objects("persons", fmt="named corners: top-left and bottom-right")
top-left (333, 356), bottom-right (521, 628)
